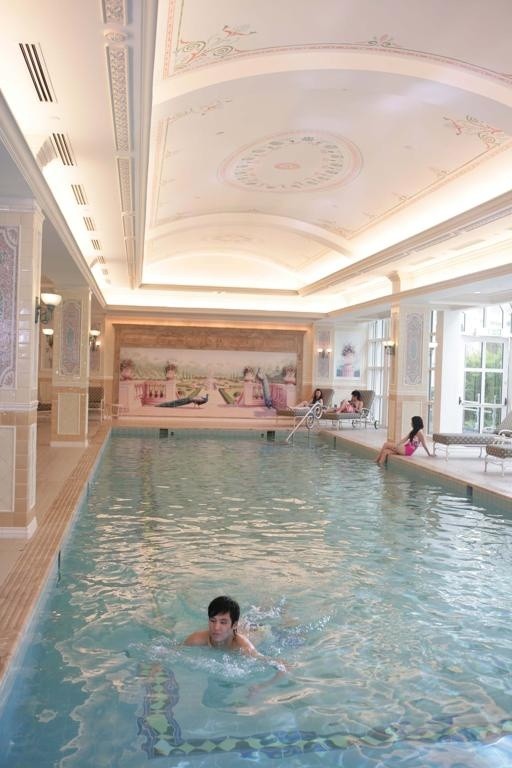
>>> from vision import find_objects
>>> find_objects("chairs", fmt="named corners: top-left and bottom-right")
top-left (322, 390), bottom-right (380, 431)
top-left (276, 388), bottom-right (333, 428)
top-left (483, 430), bottom-right (512, 476)
top-left (434, 409), bottom-right (512, 462)
top-left (99, 398), bottom-right (130, 425)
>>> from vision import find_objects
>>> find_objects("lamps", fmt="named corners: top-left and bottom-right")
top-left (317, 347), bottom-right (331, 359)
top-left (95, 341), bottom-right (101, 352)
top-left (35, 293), bottom-right (62, 324)
top-left (89, 330), bottom-right (100, 352)
top-left (42, 329), bottom-right (53, 346)
top-left (382, 340), bottom-right (394, 355)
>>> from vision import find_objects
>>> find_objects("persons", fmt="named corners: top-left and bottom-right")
top-left (373, 414), bottom-right (438, 464)
top-left (284, 386), bottom-right (324, 412)
top-left (184, 592), bottom-right (282, 665)
top-left (318, 388), bottom-right (364, 415)
